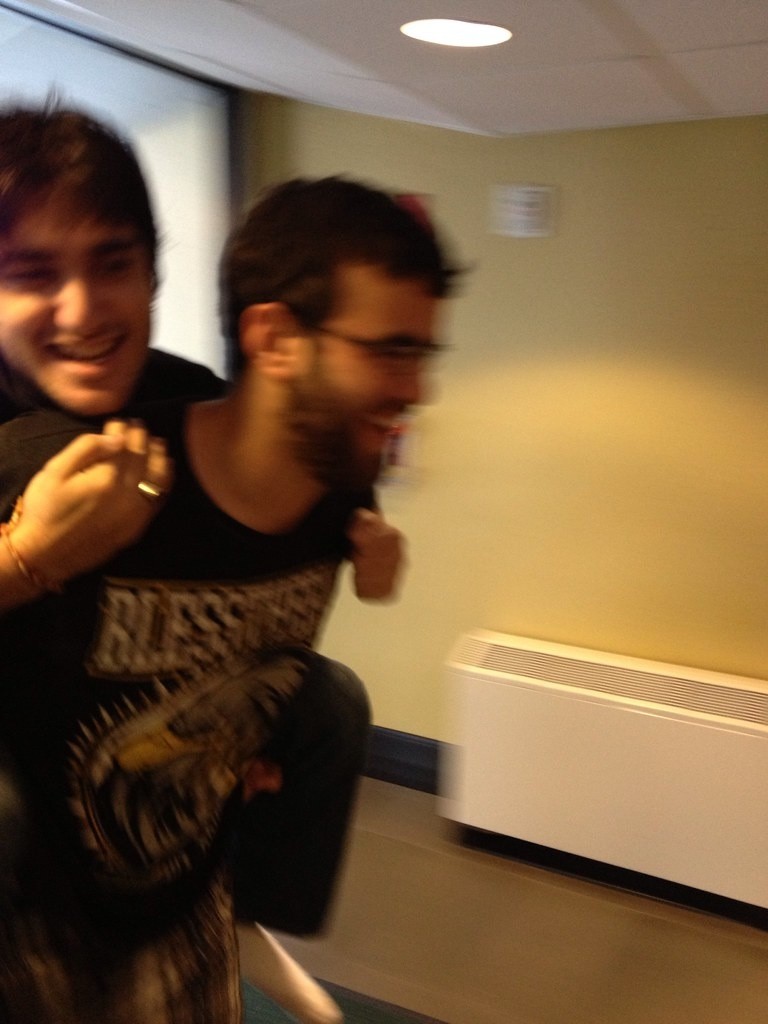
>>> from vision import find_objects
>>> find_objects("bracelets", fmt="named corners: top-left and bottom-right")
top-left (2, 521), bottom-right (56, 597)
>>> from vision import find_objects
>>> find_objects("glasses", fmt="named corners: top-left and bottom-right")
top-left (302, 322), bottom-right (452, 364)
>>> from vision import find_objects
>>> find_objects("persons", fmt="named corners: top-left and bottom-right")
top-left (0, 106), bottom-right (406, 1023)
top-left (0, 175), bottom-right (452, 1022)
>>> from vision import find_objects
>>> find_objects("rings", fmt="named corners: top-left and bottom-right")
top-left (138, 482), bottom-right (165, 501)
